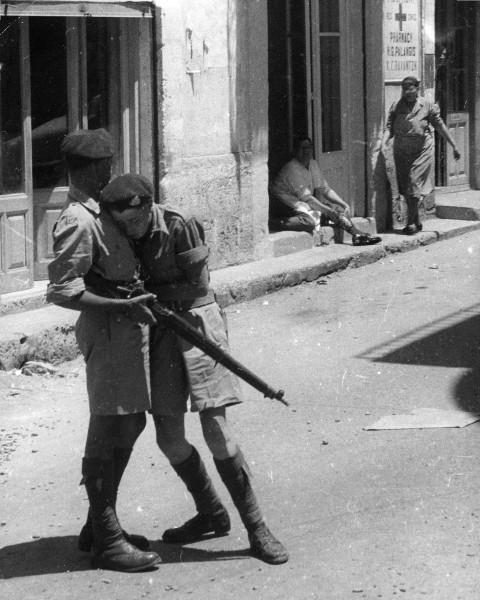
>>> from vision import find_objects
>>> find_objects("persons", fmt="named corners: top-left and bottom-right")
top-left (101, 170), bottom-right (290, 566)
top-left (45, 125), bottom-right (162, 570)
top-left (379, 75), bottom-right (459, 235)
top-left (269, 137), bottom-right (382, 246)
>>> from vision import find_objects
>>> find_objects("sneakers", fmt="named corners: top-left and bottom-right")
top-left (352, 232), bottom-right (382, 245)
top-left (402, 222), bottom-right (423, 235)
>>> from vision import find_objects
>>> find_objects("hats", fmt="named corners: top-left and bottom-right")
top-left (99, 173), bottom-right (154, 212)
top-left (61, 127), bottom-right (115, 162)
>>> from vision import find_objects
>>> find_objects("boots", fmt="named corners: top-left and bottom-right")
top-left (163, 446), bottom-right (231, 545)
top-left (77, 446), bottom-right (161, 573)
top-left (214, 446), bottom-right (289, 566)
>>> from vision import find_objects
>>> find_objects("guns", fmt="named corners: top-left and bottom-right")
top-left (85, 265), bottom-right (289, 407)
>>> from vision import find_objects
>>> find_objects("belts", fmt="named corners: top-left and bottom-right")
top-left (167, 288), bottom-right (216, 313)
